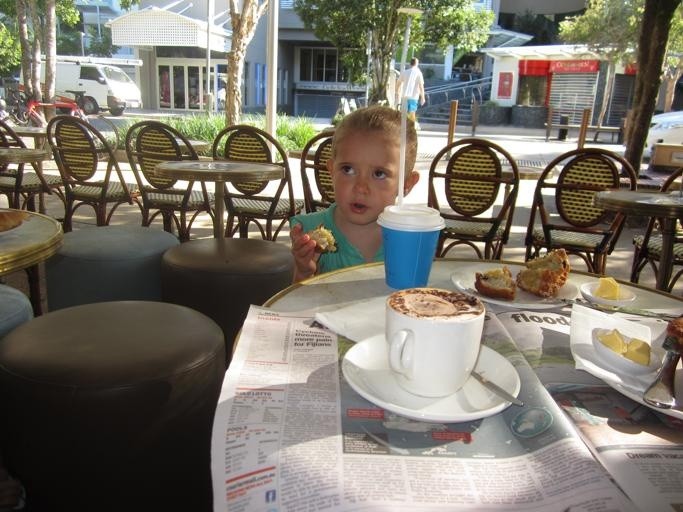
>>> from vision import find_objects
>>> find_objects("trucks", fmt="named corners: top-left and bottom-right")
top-left (19, 62), bottom-right (144, 117)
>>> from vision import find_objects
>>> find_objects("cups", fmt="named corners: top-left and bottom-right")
top-left (378, 204), bottom-right (444, 289)
top-left (386, 287), bottom-right (484, 398)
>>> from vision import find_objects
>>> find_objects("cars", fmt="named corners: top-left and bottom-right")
top-left (643, 108), bottom-right (683, 160)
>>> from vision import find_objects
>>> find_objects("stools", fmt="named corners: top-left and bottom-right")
top-left (161, 237), bottom-right (296, 334)
top-left (0, 283), bottom-right (34, 339)
top-left (46, 224), bottom-right (182, 314)
top-left (0, 300), bottom-right (227, 440)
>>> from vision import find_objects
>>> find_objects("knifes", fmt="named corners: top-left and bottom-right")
top-left (471, 372), bottom-right (524, 408)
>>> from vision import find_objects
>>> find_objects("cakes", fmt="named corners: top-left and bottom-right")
top-left (594, 277), bottom-right (620, 301)
top-left (623, 338), bottom-right (650, 366)
top-left (594, 328), bottom-right (626, 355)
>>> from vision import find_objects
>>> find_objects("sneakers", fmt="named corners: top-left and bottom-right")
top-left (414, 121), bottom-right (421, 130)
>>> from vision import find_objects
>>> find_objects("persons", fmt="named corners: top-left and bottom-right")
top-left (395, 57), bottom-right (425, 125)
top-left (289, 107), bottom-right (421, 282)
top-left (0, 98), bottom-right (18, 141)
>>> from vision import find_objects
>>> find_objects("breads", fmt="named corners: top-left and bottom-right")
top-left (306, 224), bottom-right (337, 252)
top-left (473, 265), bottom-right (515, 299)
top-left (516, 248), bottom-right (569, 297)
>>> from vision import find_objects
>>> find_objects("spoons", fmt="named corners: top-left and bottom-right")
top-left (645, 347), bottom-right (679, 411)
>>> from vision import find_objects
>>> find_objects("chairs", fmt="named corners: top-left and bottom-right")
top-left (0, 119), bottom-right (76, 226)
top-left (47, 115), bottom-right (152, 234)
top-left (525, 148), bottom-right (637, 275)
top-left (212, 125), bottom-right (304, 243)
top-left (301, 129), bottom-right (334, 215)
top-left (125, 120), bottom-right (215, 244)
top-left (630, 166), bottom-right (683, 293)
top-left (428, 138), bottom-right (520, 260)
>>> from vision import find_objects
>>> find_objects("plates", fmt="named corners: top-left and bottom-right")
top-left (589, 318), bottom-right (682, 420)
top-left (580, 282), bottom-right (636, 308)
top-left (450, 262), bottom-right (576, 308)
top-left (593, 330), bottom-right (660, 375)
top-left (342, 332), bottom-right (519, 422)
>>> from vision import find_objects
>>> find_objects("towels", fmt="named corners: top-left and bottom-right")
top-left (314, 296), bottom-right (394, 344)
top-left (570, 303), bottom-right (660, 395)
